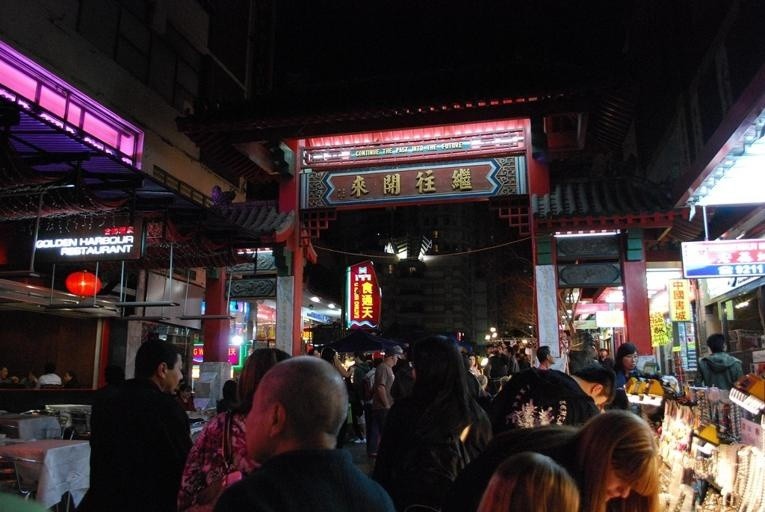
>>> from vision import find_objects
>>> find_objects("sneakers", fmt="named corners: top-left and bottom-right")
top-left (348, 435), bottom-right (367, 443)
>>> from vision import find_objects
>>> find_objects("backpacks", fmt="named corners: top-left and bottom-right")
top-left (360, 370), bottom-right (377, 401)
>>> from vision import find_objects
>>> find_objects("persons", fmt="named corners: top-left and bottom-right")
top-left (695, 332), bottom-right (744, 392)
top-left (0, 333), bottom-right (680, 512)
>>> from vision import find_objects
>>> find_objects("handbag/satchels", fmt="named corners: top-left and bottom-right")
top-left (186, 411), bottom-right (243, 512)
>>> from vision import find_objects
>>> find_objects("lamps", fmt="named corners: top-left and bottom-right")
top-left (45, 242), bottom-right (237, 322)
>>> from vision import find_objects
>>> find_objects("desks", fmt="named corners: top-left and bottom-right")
top-left (0, 403), bottom-right (92, 512)
